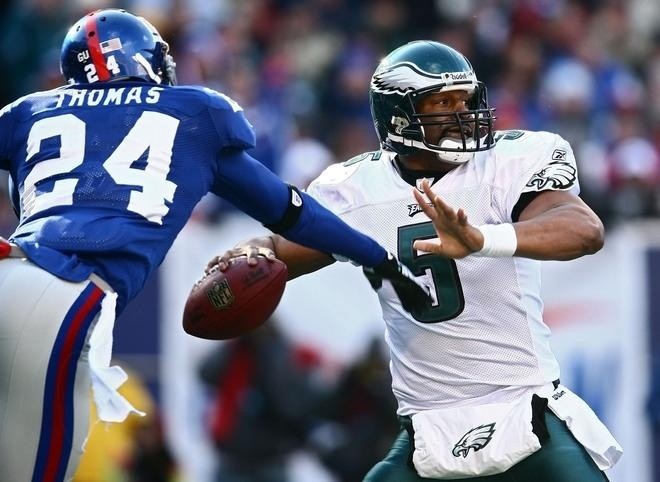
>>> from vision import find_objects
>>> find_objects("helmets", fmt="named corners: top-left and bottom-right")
top-left (60, 9), bottom-right (178, 88)
top-left (369, 40), bottom-right (498, 156)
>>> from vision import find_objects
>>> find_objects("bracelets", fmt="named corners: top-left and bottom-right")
top-left (468, 223), bottom-right (519, 258)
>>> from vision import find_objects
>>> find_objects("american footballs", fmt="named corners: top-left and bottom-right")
top-left (183, 254), bottom-right (288, 340)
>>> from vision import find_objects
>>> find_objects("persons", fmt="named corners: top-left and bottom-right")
top-left (1, 9), bottom-right (435, 482)
top-left (0, 228), bottom-right (660, 482)
top-left (201, 39), bottom-right (621, 482)
top-left (200, 321), bottom-right (402, 482)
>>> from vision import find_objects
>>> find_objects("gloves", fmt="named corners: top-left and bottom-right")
top-left (364, 251), bottom-right (437, 318)
